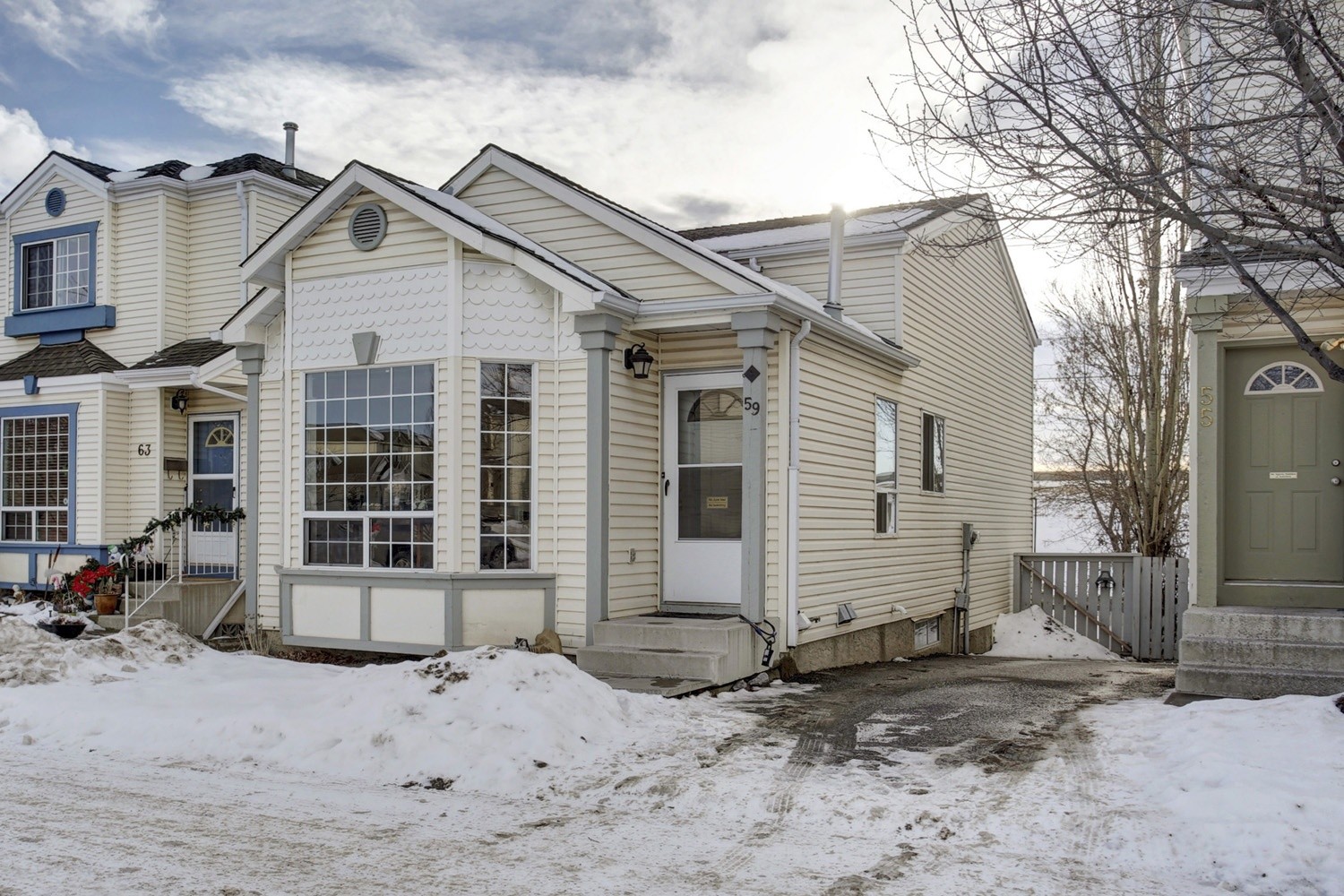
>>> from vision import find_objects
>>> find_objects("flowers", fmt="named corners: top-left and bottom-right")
top-left (70, 564), bottom-right (124, 599)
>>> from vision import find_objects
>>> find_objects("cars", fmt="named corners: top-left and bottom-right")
top-left (370, 510), bottom-right (518, 568)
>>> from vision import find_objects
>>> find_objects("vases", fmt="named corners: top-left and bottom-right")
top-left (92, 591), bottom-right (122, 616)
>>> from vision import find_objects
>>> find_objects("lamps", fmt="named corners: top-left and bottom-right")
top-left (170, 387), bottom-right (190, 416)
top-left (623, 341), bottom-right (655, 380)
